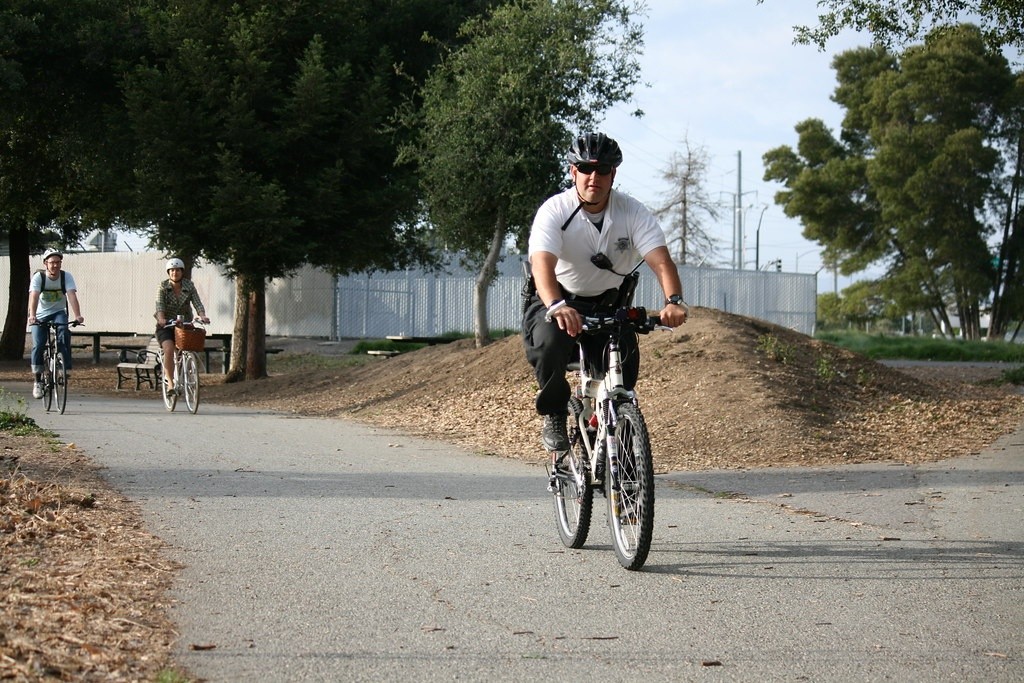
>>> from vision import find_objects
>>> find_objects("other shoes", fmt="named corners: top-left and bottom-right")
top-left (198, 382), bottom-right (205, 387)
top-left (167, 388), bottom-right (175, 397)
top-left (58, 370), bottom-right (70, 378)
top-left (32, 381), bottom-right (43, 399)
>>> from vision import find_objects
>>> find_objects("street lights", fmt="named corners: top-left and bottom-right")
top-left (742, 204), bottom-right (754, 269)
top-left (755, 206), bottom-right (769, 270)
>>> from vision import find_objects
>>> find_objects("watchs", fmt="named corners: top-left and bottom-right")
top-left (665, 294), bottom-right (684, 306)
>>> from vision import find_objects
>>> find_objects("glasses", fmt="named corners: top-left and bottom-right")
top-left (573, 163), bottom-right (613, 176)
top-left (49, 261), bottom-right (62, 265)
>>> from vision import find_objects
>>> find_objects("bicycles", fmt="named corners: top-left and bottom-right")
top-left (160, 315), bottom-right (208, 417)
top-left (546, 311), bottom-right (677, 571)
top-left (29, 320), bottom-right (86, 417)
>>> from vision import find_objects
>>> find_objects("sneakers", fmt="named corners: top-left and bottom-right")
top-left (542, 410), bottom-right (570, 451)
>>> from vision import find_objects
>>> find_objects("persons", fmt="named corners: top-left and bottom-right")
top-left (153, 258), bottom-right (209, 397)
top-left (520, 131), bottom-right (688, 528)
top-left (28, 247), bottom-right (83, 398)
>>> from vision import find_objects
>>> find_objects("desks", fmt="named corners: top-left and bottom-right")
top-left (207, 333), bottom-right (269, 373)
top-left (69, 331), bottom-right (137, 361)
top-left (386, 336), bottom-right (463, 346)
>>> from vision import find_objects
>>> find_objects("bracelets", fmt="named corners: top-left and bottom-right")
top-left (548, 299), bottom-right (561, 306)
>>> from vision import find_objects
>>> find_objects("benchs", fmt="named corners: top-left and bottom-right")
top-left (367, 350), bottom-right (402, 356)
top-left (43, 343), bottom-right (91, 349)
top-left (200, 347), bottom-right (286, 373)
top-left (116, 336), bottom-right (161, 390)
top-left (101, 344), bottom-right (147, 362)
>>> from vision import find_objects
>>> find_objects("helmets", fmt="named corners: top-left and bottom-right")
top-left (43, 248), bottom-right (63, 264)
top-left (567, 133), bottom-right (623, 166)
top-left (166, 257), bottom-right (185, 274)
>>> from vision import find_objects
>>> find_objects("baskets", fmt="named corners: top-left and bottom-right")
top-left (174, 322), bottom-right (206, 352)
